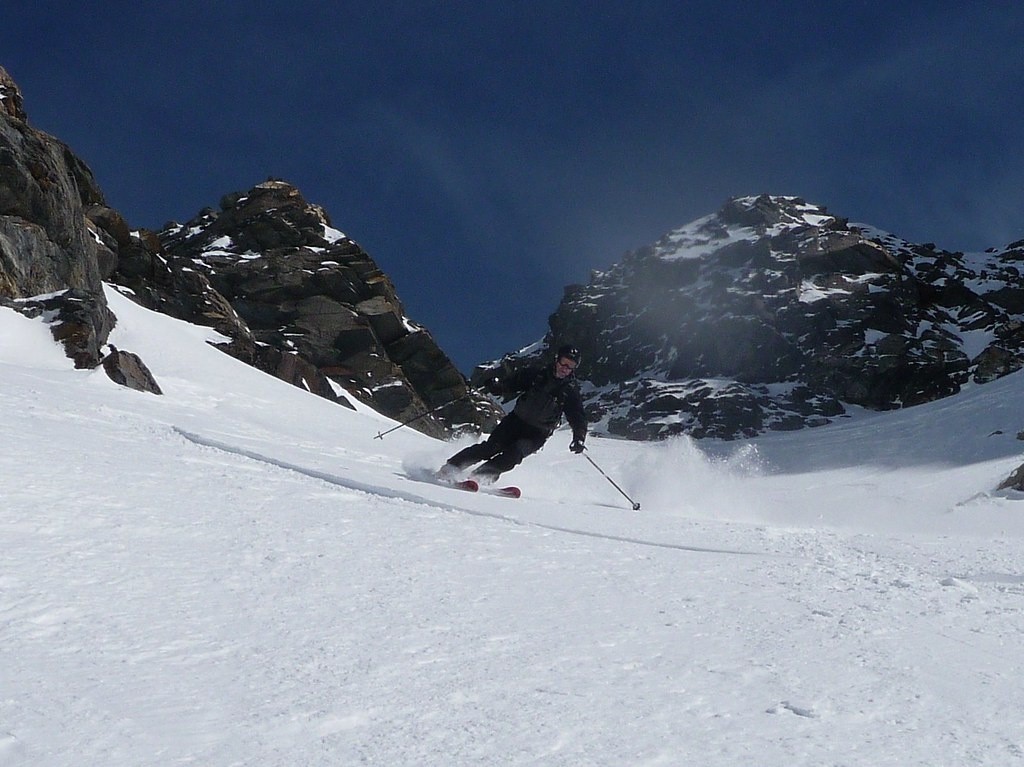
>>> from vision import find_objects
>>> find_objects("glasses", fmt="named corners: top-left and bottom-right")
top-left (558, 357), bottom-right (577, 369)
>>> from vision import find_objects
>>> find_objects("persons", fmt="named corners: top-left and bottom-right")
top-left (433, 344), bottom-right (588, 487)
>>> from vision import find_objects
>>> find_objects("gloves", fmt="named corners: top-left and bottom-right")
top-left (569, 439), bottom-right (585, 454)
top-left (486, 377), bottom-right (503, 396)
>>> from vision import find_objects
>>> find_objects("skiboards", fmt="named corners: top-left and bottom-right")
top-left (427, 479), bottom-right (478, 492)
top-left (482, 485), bottom-right (522, 498)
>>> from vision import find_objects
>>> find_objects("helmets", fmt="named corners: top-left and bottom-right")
top-left (559, 346), bottom-right (581, 368)
